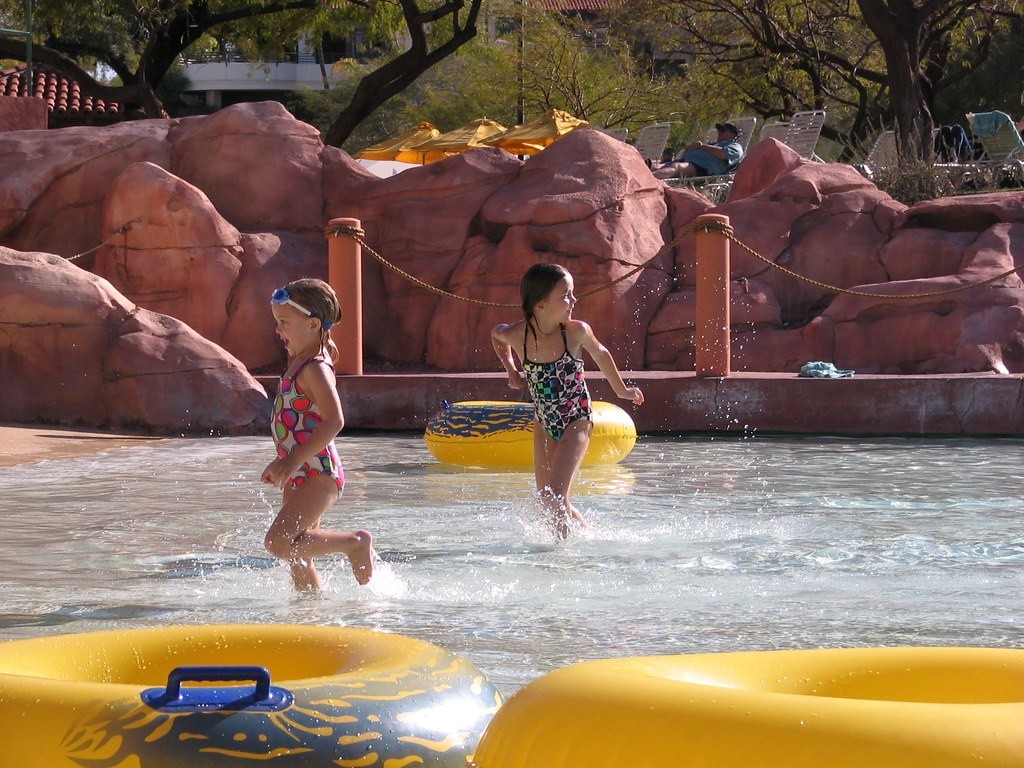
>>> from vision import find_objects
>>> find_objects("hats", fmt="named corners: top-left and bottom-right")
top-left (715, 121), bottom-right (739, 139)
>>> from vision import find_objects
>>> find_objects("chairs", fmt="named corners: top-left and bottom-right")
top-left (965, 111), bottom-right (1024, 168)
top-left (864, 128), bottom-right (938, 171)
top-left (605, 110), bottom-right (826, 203)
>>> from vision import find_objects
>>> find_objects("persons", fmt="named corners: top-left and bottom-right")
top-left (259, 279), bottom-right (371, 596)
top-left (491, 264), bottom-right (645, 540)
top-left (647, 122), bottom-right (744, 179)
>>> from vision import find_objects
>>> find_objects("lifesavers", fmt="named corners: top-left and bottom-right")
top-left (467, 645), bottom-right (1024, 768)
top-left (0, 622), bottom-right (508, 768)
top-left (422, 399), bottom-right (638, 467)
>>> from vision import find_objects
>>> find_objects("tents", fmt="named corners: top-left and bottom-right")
top-left (352, 108), bottom-right (588, 166)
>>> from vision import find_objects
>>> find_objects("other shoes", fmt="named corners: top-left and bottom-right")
top-left (951, 125), bottom-right (972, 163)
top-left (934, 126), bottom-right (950, 163)
top-left (645, 157), bottom-right (652, 168)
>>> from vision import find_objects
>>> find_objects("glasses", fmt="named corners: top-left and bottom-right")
top-left (271, 287), bottom-right (312, 316)
